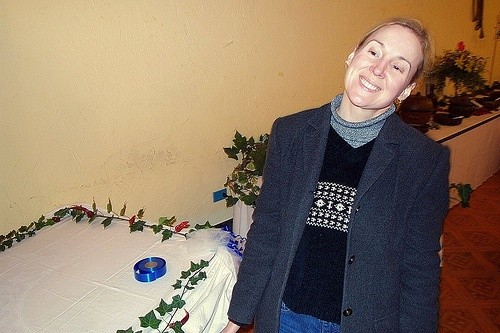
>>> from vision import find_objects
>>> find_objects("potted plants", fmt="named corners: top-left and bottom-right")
top-left (222, 128), bottom-right (271, 242)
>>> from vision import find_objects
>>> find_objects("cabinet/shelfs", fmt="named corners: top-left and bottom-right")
top-left (413, 109), bottom-right (500, 214)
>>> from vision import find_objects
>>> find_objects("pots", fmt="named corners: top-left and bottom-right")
top-left (399, 92), bottom-right (435, 123)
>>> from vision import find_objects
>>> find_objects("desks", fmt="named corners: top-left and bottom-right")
top-left (0, 202), bottom-right (244, 333)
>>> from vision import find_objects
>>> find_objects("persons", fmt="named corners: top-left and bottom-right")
top-left (227, 16), bottom-right (454, 333)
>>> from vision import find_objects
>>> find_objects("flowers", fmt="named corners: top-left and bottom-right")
top-left (432, 38), bottom-right (490, 98)
